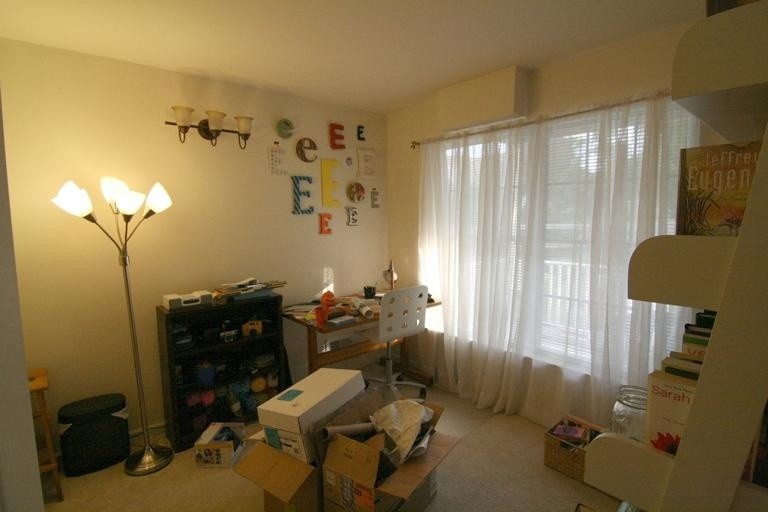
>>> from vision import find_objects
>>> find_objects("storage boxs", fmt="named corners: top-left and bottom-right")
top-left (194, 366), bottom-right (461, 511)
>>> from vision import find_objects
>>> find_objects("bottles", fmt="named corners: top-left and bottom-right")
top-left (611, 384), bottom-right (649, 442)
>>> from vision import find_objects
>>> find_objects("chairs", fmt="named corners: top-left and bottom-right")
top-left (364, 284), bottom-right (429, 402)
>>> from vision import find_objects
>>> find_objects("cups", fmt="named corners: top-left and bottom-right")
top-left (364, 285), bottom-right (376, 299)
top-left (314, 306), bottom-right (328, 325)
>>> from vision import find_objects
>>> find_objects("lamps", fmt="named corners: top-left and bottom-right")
top-left (50, 175), bottom-right (173, 476)
top-left (162, 104), bottom-right (254, 152)
top-left (382, 259), bottom-right (399, 293)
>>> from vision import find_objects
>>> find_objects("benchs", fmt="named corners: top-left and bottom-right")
top-left (283, 287), bottom-right (442, 386)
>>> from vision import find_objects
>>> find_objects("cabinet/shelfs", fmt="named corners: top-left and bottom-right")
top-left (155, 290), bottom-right (282, 452)
top-left (574, 2), bottom-right (768, 511)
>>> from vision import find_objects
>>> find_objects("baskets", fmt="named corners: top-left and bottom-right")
top-left (540, 413), bottom-right (611, 484)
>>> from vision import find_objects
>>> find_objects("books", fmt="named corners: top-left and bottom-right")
top-left (328, 314), bottom-right (356, 328)
top-left (556, 429), bottom-right (590, 441)
top-left (638, 306), bottom-right (767, 484)
top-left (552, 424), bottom-right (585, 438)
top-left (672, 138), bottom-right (763, 237)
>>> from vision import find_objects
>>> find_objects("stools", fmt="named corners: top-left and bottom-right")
top-left (26, 366), bottom-right (65, 501)
